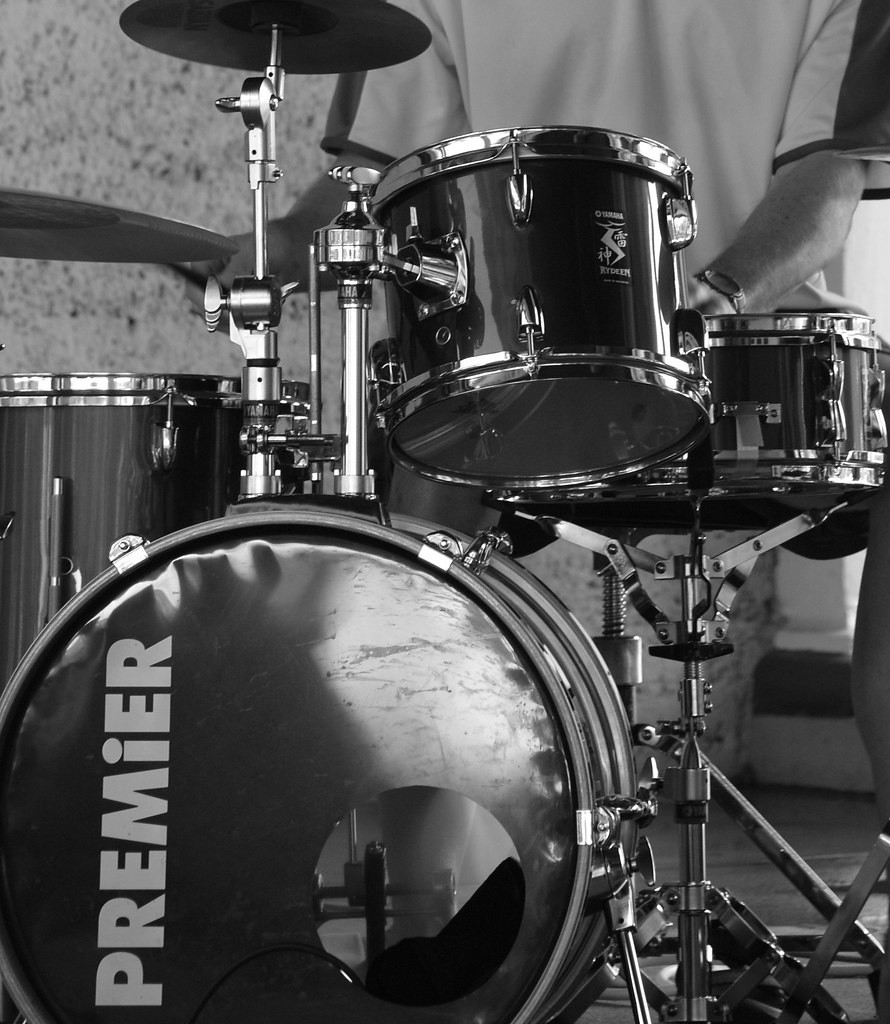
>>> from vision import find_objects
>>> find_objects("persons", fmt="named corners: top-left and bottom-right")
top-left (185, 0), bottom-right (890, 1024)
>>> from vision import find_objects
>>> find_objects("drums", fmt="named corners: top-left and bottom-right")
top-left (368, 122), bottom-right (712, 489)
top-left (0, 366), bottom-right (310, 696)
top-left (0, 495), bottom-right (647, 1024)
top-left (690, 310), bottom-right (889, 496)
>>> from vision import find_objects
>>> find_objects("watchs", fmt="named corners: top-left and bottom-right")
top-left (694, 269), bottom-right (748, 315)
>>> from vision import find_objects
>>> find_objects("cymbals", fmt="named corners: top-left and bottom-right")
top-left (0, 190), bottom-right (241, 266)
top-left (118, 0), bottom-right (432, 74)
